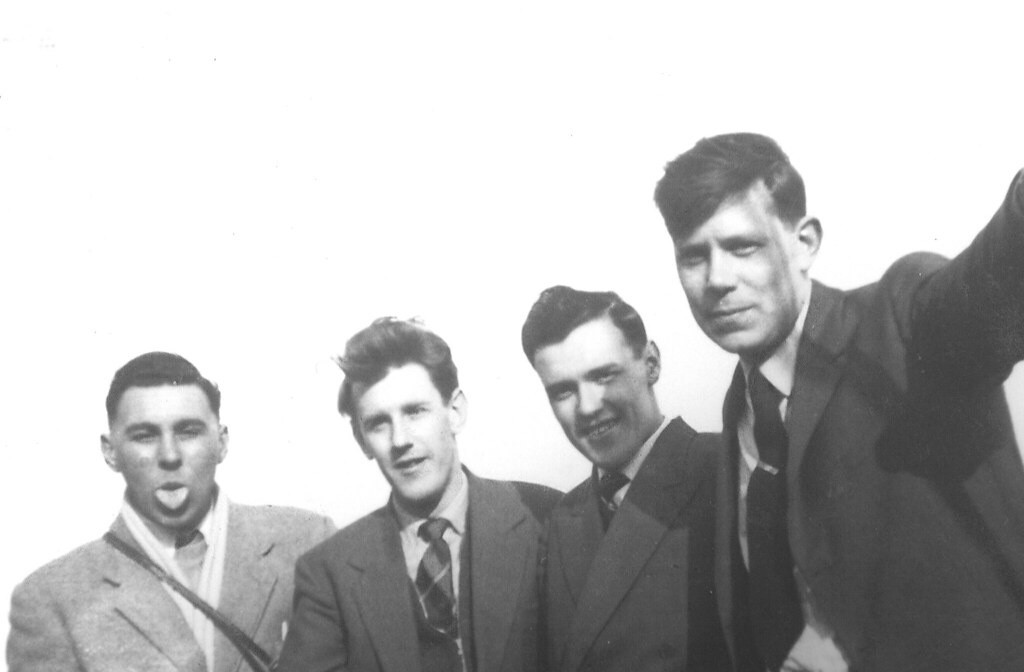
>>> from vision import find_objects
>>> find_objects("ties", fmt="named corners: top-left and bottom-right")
top-left (411, 517), bottom-right (460, 639)
top-left (595, 467), bottom-right (625, 529)
top-left (746, 364), bottom-right (804, 671)
top-left (174, 531), bottom-right (209, 618)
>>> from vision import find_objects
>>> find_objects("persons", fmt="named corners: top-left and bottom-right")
top-left (5, 350), bottom-right (338, 672)
top-left (279, 318), bottom-right (569, 671)
top-left (653, 133), bottom-right (1024, 671)
top-left (522, 287), bottom-right (723, 671)
